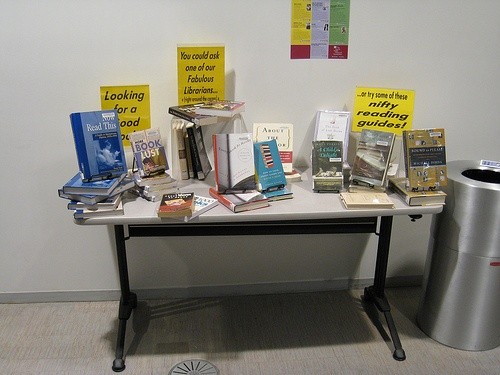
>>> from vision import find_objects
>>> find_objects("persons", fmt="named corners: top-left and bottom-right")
top-left (101, 138), bottom-right (124, 171)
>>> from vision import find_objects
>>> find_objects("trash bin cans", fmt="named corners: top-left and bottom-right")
top-left (416, 159), bottom-right (500, 352)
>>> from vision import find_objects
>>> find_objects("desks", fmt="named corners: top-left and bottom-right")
top-left (73, 167), bottom-right (443, 372)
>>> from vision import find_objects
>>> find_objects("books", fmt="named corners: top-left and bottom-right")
top-left (208, 122), bottom-right (302, 213)
top-left (157, 190), bottom-right (219, 221)
top-left (58, 170), bottom-right (136, 219)
top-left (129, 127), bottom-right (171, 179)
top-left (127, 168), bottom-right (180, 202)
top-left (195, 98), bottom-right (247, 117)
top-left (402, 128), bottom-right (448, 193)
top-left (170, 114), bottom-right (213, 180)
top-left (388, 174), bottom-right (447, 206)
top-left (340, 191), bottom-right (395, 210)
top-left (157, 192), bottom-right (195, 218)
top-left (168, 102), bottom-right (237, 126)
top-left (69, 108), bottom-right (129, 183)
top-left (310, 110), bottom-right (400, 194)
